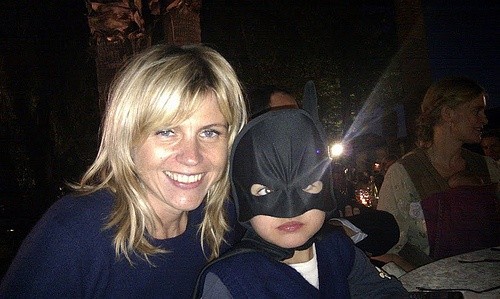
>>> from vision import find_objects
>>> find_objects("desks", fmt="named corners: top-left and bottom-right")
top-left (397, 246), bottom-right (500, 299)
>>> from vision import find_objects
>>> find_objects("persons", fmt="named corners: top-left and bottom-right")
top-left (332, 129), bottom-right (499, 209)
top-left (246, 87), bottom-right (401, 257)
top-left (369, 75), bottom-right (500, 273)
top-left (192, 105), bottom-right (414, 299)
top-left (0, 43), bottom-right (248, 299)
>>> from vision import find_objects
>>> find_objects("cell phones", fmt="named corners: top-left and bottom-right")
top-left (409, 292), bottom-right (464, 299)
